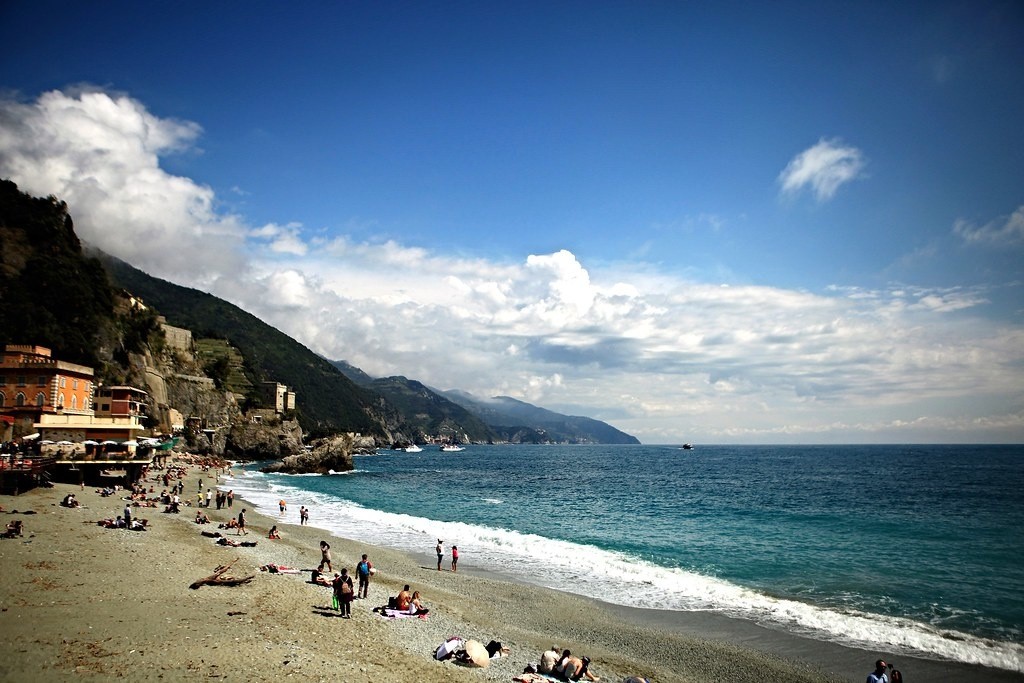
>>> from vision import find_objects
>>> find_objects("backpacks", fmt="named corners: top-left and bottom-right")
top-left (339, 575), bottom-right (351, 594)
top-left (359, 561), bottom-right (369, 577)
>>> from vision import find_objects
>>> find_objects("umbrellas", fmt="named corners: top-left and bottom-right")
top-left (465, 640), bottom-right (490, 667)
top-left (21, 432), bottom-right (160, 454)
top-left (436, 637), bottom-right (462, 660)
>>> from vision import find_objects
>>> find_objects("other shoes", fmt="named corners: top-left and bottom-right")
top-left (340, 614), bottom-right (345, 617)
top-left (347, 614), bottom-right (351, 618)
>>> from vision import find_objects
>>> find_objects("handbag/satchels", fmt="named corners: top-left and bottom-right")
top-left (333, 595), bottom-right (338, 610)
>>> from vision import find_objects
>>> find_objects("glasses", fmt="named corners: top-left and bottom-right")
top-left (879, 666), bottom-right (887, 669)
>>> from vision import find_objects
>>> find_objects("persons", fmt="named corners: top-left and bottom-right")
top-left (890, 670), bottom-right (902, 683)
top-left (450, 545), bottom-right (459, 572)
top-left (866, 659), bottom-right (889, 683)
top-left (540, 644), bottom-right (561, 674)
top-left (565, 657), bottom-right (600, 681)
top-left (436, 538), bottom-right (444, 570)
top-left (95, 452), bottom-right (430, 621)
top-left (554, 649), bottom-right (571, 678)
top-left (0, 441), bottom-right (86, 539)
top-left (455, 639), bottom-right (509, 663)
